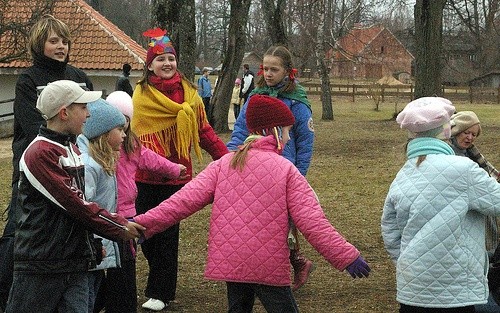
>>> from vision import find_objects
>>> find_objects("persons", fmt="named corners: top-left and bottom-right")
top-left (4, 79), bottom-right (146, 313)
top-left (450, 110), bottom-right (500, 183)
top-left (198, 69), bottom-right (212, 112)
top-left (226, 46), bottom-right (316, 292)
top-left (131, 27), bottom-right (231, 310)
top-left (232, 78), bottom-right (242, 120)
top-left (76, 97), bottom-right (127, 313)
top-left (381, 97), bottom-right (500, 313)
top-left (0, 15), bottom-right (94, 313)
top-left (240, 64), bottom-right (255, 103)
top-left (106, 91), bottom-right (191, 313)
top-left (116, 64), bottom-right (133, 98)
top-left (128, 94), bottom-right (371, 313)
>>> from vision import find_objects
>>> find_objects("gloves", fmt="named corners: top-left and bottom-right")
top-left (346, 257), bottom-right (371, 279)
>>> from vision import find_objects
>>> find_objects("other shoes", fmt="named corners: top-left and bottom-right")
top-left (141, 298), bottom-right (173, 311)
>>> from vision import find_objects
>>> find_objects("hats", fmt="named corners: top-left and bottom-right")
top-left (246, 95), bottom-right (295, 131)
top-left (235, 78), bottom-right (241, 85)
top-left (395, 97), bottom-right (455, 131)
top-left (142, 28), bottom-right (176, 65)
top-left (122, 64), bottom-right (131, 73)
top-left (452, 111), bottom-right (480, 135)
top-left (105, 90), bottom-right (133, 120)
top-left (82, 95), bottom-right (125, 139)
top-left (36, 80), bottom-right (102, 120)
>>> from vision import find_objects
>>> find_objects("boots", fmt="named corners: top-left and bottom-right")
top-left (289, 248), bottom-right (312, 292)
